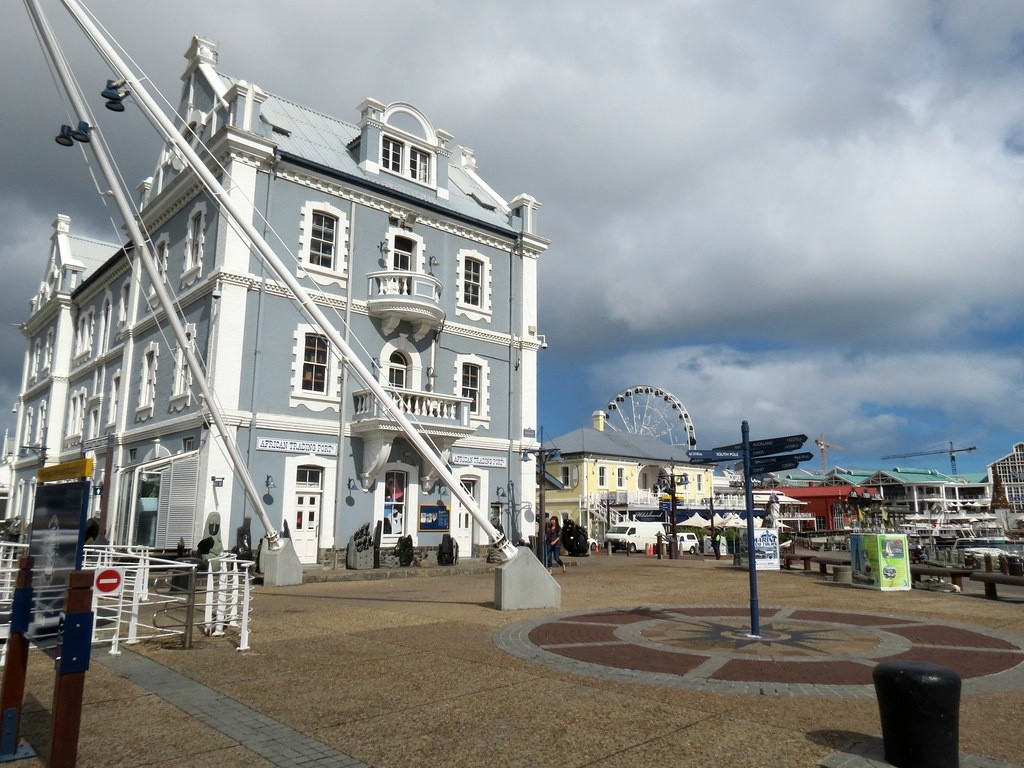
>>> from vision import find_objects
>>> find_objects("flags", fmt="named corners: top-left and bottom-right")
top-left (859, 508), bottom-right (866, 521)
top-left (882, 509), bottom-right (888, 520)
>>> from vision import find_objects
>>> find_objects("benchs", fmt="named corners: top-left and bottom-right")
top-left (910, 567), bottom-right (972, 587)
top-left (810, 556), bottom-right (852, 574)
top-left (783, 553), bottom-right (815, 571)
top-left (969, 571), bottom-right (1024, 601)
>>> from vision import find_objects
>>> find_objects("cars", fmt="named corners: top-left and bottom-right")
top-left (587, 536), bottom-right (598, 550)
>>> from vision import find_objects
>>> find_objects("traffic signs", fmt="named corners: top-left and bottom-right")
top-left (686, 434), bottom-right (815, 476)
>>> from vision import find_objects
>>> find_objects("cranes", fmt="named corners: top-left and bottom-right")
top-left (815, 434), bottom-right (845, 470)
top-left (881, 442), bottom-right (977, 475)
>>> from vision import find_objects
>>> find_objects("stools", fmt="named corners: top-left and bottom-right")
top-left (833, 565), bottom-right (852, 582)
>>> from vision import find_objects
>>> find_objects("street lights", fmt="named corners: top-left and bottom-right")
top-left (519, 426), bottom-right (562, 572)
top-left (656, 458), bottom-right (690, 559)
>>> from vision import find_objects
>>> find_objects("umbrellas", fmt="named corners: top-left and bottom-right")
top-left (676, 513), bottom-right (757, 534)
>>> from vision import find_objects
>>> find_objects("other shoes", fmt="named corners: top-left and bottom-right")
top-left (549, 568), bottom-right (552, 575)
top-left (563, 565), bottom-right (566, 573)
top-left (716, 555), bottom-right (720, 559)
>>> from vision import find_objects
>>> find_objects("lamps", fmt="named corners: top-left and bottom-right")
top-left (91, 482), bottom-right (104, 497)
top-left (348, 478), bottom-right (356, 491)
top-left (497, 486), bottom-right (506, 497)
top-left (211, 476), bottom-right (225, 487)
top-left (264, 474), bottom-right (276, 489)
top-left (429, 256), bottom-right (440, 276)
top-left (438, 483), bottom-right (449, 496)
top-left (17, 445), bottom-right (51, 464)
top-left (377, 241), bottom-right (390, 254)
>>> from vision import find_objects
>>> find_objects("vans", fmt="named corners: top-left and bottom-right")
top-left (666, 532), bottom-right (700, 554)
top-left (605, 521), bottom-right (667, 552)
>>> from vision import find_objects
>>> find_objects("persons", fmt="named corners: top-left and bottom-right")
top-left (710, 528), bottom-right (721, 559)
top-left (4, 516), bottom-right (22, 559)
top-left (545, 516), bottom-right (566, 575)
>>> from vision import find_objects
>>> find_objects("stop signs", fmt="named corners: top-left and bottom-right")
top-left (92, 566), bottom-right (125, 596)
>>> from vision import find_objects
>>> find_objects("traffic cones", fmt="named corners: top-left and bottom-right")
top-left (649, 544), bottom-right (654, 556)
